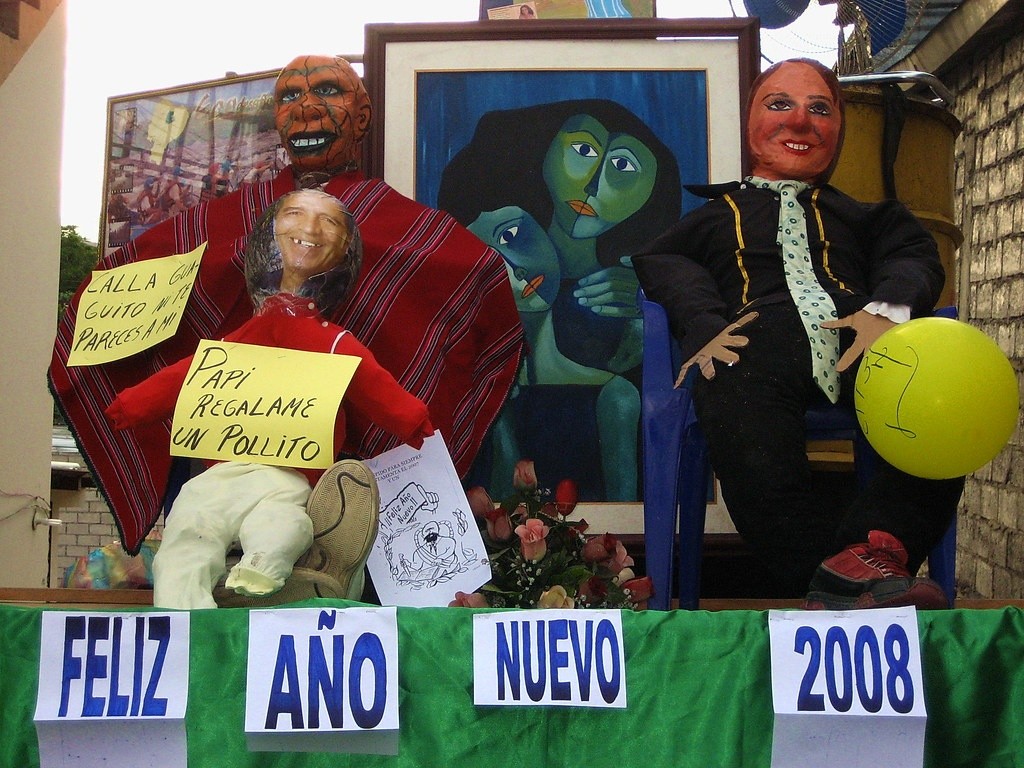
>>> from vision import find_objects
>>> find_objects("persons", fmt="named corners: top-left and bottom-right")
top-left (139, 150), bottom-right (273, 223)
top-left (105, 189), bottom-right (436, 610)
top-left (257, 189), bottom-right (354, 294)
top-left (629, 57), bottom-right (965, 609)
top-left (46, 52), bottom-right (528, 606)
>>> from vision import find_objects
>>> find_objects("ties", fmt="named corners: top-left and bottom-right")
top-left (745, 175), bottom-right (840, 403)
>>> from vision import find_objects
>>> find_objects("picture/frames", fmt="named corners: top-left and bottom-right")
top-left (360, 17), bottom-right (762, 610)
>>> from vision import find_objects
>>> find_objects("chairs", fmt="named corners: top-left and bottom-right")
top-left (636, 285), bottom-right (960, 611)
top-left (211, 460), bottom-right (381, 609)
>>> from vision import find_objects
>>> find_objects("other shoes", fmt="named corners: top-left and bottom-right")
top-left (212, 558), bottom-right (346, 608)
top-left (307, 458), bottom-right (381, 602)
top-left (804, 529), bottom-right (951, 611)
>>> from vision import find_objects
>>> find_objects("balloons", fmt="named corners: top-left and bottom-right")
top-left (854, 317), bottom-right (1019, 480)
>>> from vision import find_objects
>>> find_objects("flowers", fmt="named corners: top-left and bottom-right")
top-left (449, 455), bottom-right (655, 610)
